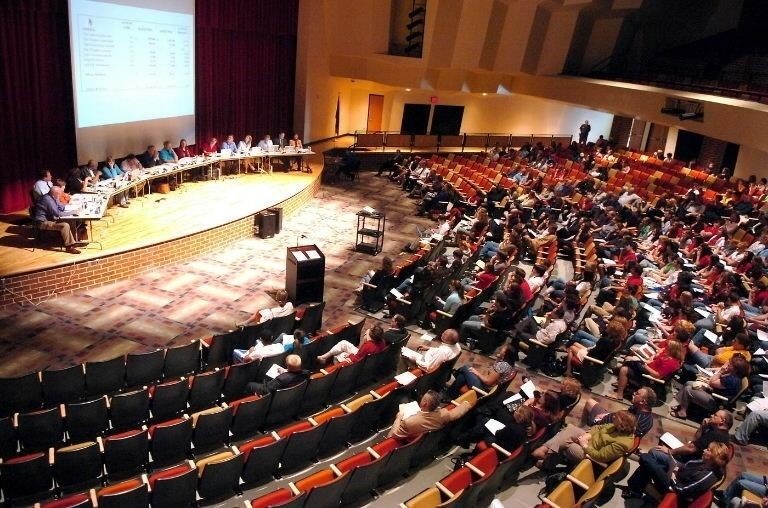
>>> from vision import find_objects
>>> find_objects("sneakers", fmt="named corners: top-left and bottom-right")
top-left (439, 367), bottom-right (457, 404)
top-left (419, 317), bottom-right (431, 330)
top-left (604, 382), bottom-right (625, 402)
top-left (121, 202), bottom-right (131, 208)
top-left (65, 246), bottom-right (81, 254)
top-left (734, 410), bottom-right (745, 421)
top-left (503, 330), bottom-right (516, 341)
top-left (317, 356), bottom-right (327, 365)
top-left (375, 170), bottom-right (427, 217)
top-left (72, 242), bottom-right (89, 248)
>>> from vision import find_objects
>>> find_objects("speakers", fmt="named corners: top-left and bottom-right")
top-left (267, 207), bottom-right (282, 234)
top-left (259, 212), bottom-right (276, 238)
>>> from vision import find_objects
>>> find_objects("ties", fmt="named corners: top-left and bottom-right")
top-left (47, 182), bottom-right (51, 188)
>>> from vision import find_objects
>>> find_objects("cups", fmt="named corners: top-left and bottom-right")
top-left (82, 145), bottom-right (311, 216)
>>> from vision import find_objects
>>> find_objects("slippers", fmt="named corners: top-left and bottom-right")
top-left (670, 405), bottom-right (686, 420)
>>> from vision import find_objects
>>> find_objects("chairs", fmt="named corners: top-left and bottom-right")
top-left (325, 153), bottom-right (360, 186)
top-left (352, 269), bottom-right (396, 313)
top-left (67, 146), bottom-right (212, 208)
top-left (385, 137), bottom-right (764, 430)
top-left (26, 190), bottom-right (110, 250)
top-left (198, 141), bottom-right (317, 180)
top-left (1, 301), bottom-right (764, 506)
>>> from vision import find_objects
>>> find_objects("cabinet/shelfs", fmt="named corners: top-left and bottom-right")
top-left (285, 244), bottom-right (325, 308)
top-left (356, 206), bottom-right (386, 256)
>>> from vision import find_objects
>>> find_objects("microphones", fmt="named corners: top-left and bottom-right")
top-left (297, 234), bottom-right (306, 247)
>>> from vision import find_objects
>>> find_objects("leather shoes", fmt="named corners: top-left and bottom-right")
top-left (244, 167), bottom-right (256, 174)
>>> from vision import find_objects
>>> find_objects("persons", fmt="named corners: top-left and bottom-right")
top-left (119, 152), bottom-right (147, 196)
top-left (31, 157), bottom-right (103, 206)
top-left (199, 137), bottom-right (219, 180)
top-left (140, 145), bottom-right (163, 188)
top-left (32, 185), bottom-right (82, 255)
top-left (177, 138), bottom-right (206, 182)
top-left (578, 120), bottom-right (591, 145)
top-left (159, 140), bottom-right (179, 164)
top-left (221, 134), bottom-right (237, 175)
top-left (101, 155), bottom-right (131, 209)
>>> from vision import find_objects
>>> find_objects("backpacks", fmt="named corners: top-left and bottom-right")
top-left (544, 470), bottom-right (567, 494)
